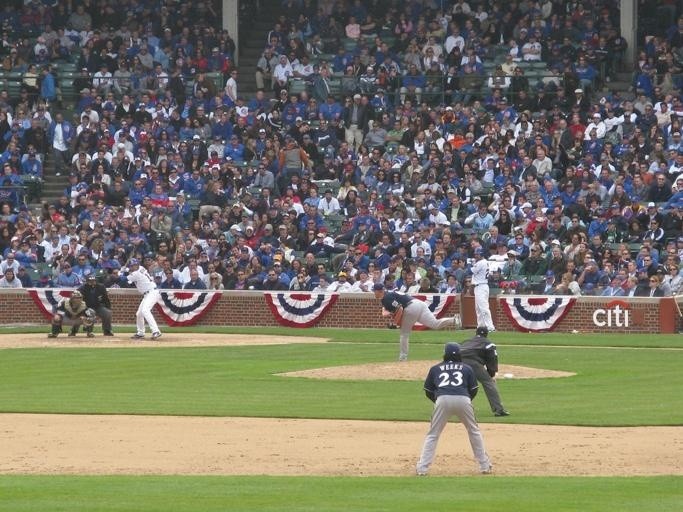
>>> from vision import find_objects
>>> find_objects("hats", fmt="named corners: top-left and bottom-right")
top-left (370, 282), bottom-right (383, 290)
top-left (445, 343), bottom-right (460, 355)
top-left (477, 326), bottom-right (488, 334)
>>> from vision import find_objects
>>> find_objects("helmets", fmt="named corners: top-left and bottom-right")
top-left (474, 248), bottom-right (484, 257)
top-left (128, 258), bottom-right (140, 270)
top-left (87, 273), bottom-right (96, 283)
top-left (70, 291), bottom-right (82, 304)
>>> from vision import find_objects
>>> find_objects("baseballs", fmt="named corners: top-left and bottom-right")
top-left (249, 286), bottom-right (254, 290)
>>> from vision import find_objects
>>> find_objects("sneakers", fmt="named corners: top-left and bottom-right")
top-left (495, 410), bottom-right (510, 416)
top-left (48, 331), bottom-right (162, 340)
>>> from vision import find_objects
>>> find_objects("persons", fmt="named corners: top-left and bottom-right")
top-left (457, 325), bottom-right (512, 416)
top-left (416, 342), bottom-right (492, 476)
top-left (117, 258), bottom-right (163, 341)
top-left (1, 0), bottom-right (682, 296)
top-left (47, 291), bottom-right (96, 339)
top-left (371, 282), bottom-right (462, 361)
top-left (463, 250), bottom-right (497, 331)
top-left (67, 273), bottom-right (114, 337)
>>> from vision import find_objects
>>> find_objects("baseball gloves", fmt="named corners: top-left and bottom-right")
top-left (84, 313), bottom-right (97, 326)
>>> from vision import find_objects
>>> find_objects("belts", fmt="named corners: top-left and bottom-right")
top-left (144, 287), bottom-right (156, 295)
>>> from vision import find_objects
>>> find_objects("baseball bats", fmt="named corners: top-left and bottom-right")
top-left (120, 252), bottom-right (134, 272)
